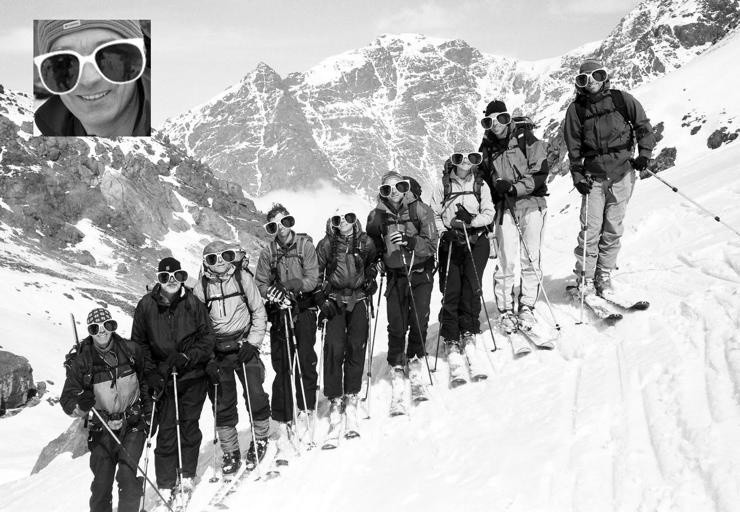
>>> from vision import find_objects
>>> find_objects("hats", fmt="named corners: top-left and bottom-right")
top-left (202, 241), bottom-right (229, 255)
top-left (579, 58), bottom-right (604, 74)
top-left (482, 99), bottom-right (507, 117)
top-left (36, 19), bottom-right (147, 56)
top-left (453, 141), bottom-right (475, 153)
top-left (86, 307), bottom-right (113, 326)
top-left (157, 256), bottom-right (182, 273)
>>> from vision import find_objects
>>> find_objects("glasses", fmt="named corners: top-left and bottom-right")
top-left (87, 319), bottom-right (118, 337)
top-left (480, 112), bottom-right (512, 130)
top-left (449, 151), bottom-right (485, 166)
top-left (574, 68), bottom-right (609, 88)
top-left (330, 212), bottom-right (358, 228)
top-left (31, 36), bottom-right (148, 96)
top-left (262, 215), bottom-right (296, 236)
top-left (378, 179), bottom-right (411, 198)
top-left (203, 249), bottom-right (237, 267)
top-left (155, 269), bottom-right (189, 286)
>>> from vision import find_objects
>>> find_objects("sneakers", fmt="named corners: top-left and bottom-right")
top-left (593, 267), bottom-right (616, 296)
top-left (328, 398), bottom-right (344, 425)
top-left (390, 364), bottom-right (406, 390)
top-left (498, 311), bottom-right (518, 334)
top-left (344, 393), bottom-right (359, 416)
top-left (444, 339), bottom-right (461, 368)
top-left (221, 448), bottom-right (242, 475)
top-left (577, 276), bottom-right (597, 300)
top-left (155, 488), bottom-right (176, 504)
top-left (246, 438), bottom-right (268, 462)
top-left (517, 305), bottom-right (540, 331)
top-left (178, 477), bottom-right (195, 494)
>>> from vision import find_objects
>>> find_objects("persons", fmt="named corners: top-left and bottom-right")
top-left (191, 242), bottom-right (268, 474)
top-left (315, 205), bottom-right (379, 416)
top-left (255, 202), bottom-right (320, 435)
top-left (430, 141), bottom-right (498, 350)
top-left (565, 62), bottom-right (657, 293)
top-left (366, 172), bottom-right (438, 379)
top-left (131, 256), bottom-right (217, 505)
top-left (477, 99), bottom-right (549, 333)
top-left (33, 20), bottom-right (152, 137)
top-left (59, 309), bottom-right (151, 512)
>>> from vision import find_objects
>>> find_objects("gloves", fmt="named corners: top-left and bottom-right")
top-left (442, 228), bottom-right (465, 242)
top-left (145, 372), bottom-right (167, 401)
top-left (236, 340), bottom-right (258, 366)
top-left (204, 359), bottom-right (225, 385)
top-left (364, 278), bottom-right (378, 298)
top-left (454, 209), bottom-right (473, 225)
top-left (374, 258), bottom-right (386, 274)
top-left (75, 391), bottom-right (97, 413)
top-left (631, 156), bottom-right (650, 172)
top-left (174, 350), bottom-right (192, 375)
top-left (319, 298), bottom-right (338, 321)
top-left (266, 283), bottom-right (298, 310)
top-left (389, 230), bottom-right (417, 252)
top-left (495, 179), bottom-right (512, 194)
top-left (573, 177), bottom-right (595, 196)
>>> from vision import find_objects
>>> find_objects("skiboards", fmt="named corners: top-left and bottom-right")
top-left (148, 396), bottom-right (360, 511)
top-left (565, 283), bottom-right (650, 321)
top-left (503, 316), bottom-right (555, 354)
top-left (443, 344), bottom-right (487, 387)
top-left (389, 358), bottom-right (430, 416)
